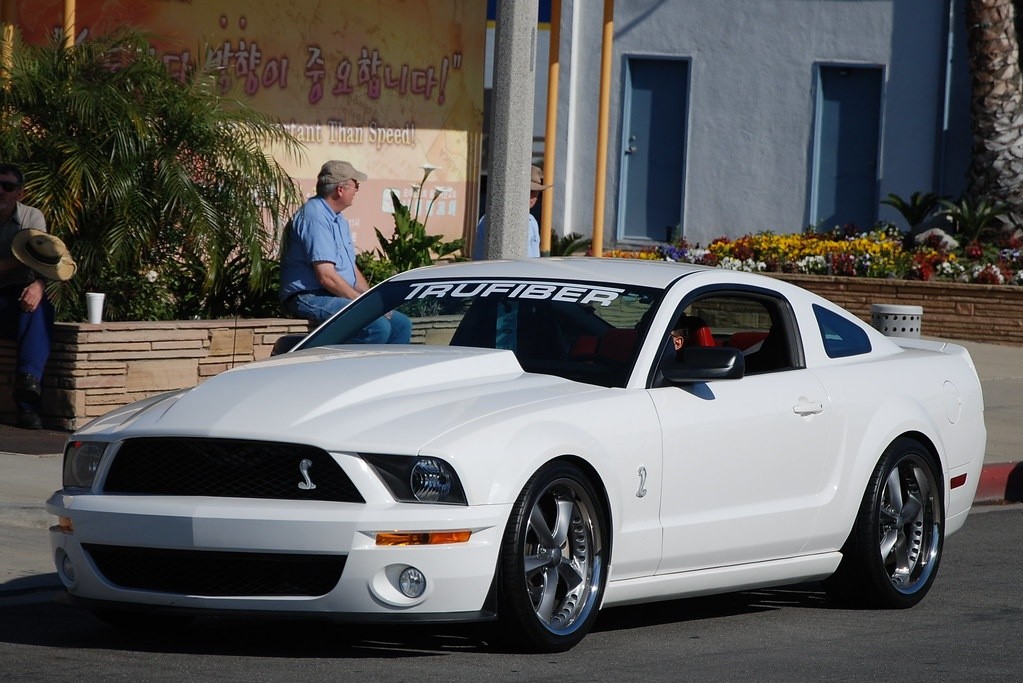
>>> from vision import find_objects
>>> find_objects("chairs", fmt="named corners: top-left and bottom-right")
top-left (572, 318), bottom-right (772, 367)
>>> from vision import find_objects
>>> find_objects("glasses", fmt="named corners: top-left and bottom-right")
top-left (343, 177), bottom-right (361, 192)
top-left (635, 322), bottom-right (677, 340)
top-left (0, 181), bottom-right (17, 192)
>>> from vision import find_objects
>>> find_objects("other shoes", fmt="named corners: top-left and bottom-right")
top-left (17, 407), bottom-right (43, 429)
top-left (12, 373), bottom-right (41, 402)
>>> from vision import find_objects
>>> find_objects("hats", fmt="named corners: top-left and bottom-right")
top-left (529, 164), bottom-right (553, 191)
top-left (318, 160), bottom-right (367, 183)
top-left (11, 227), bottom-right (78, 281)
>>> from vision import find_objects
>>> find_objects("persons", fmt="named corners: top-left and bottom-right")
top-left (279, 159), bottom-right (413, 347)
top-left (471, 164), bottom-right (555, 260)
top-left (0, 161), bottom-right (79, 430)
top-left (634, 309), bottom-right (689, 350)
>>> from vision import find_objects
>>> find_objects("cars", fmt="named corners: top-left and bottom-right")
top-left (41, 257), bottom-right (988, 656)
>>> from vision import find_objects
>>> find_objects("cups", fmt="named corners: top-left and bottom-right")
top-left (85, 292), bottom-right (105, 324)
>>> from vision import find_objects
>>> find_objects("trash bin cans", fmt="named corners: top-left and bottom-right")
top-left (871, 302), bottom-right (923, 341)
